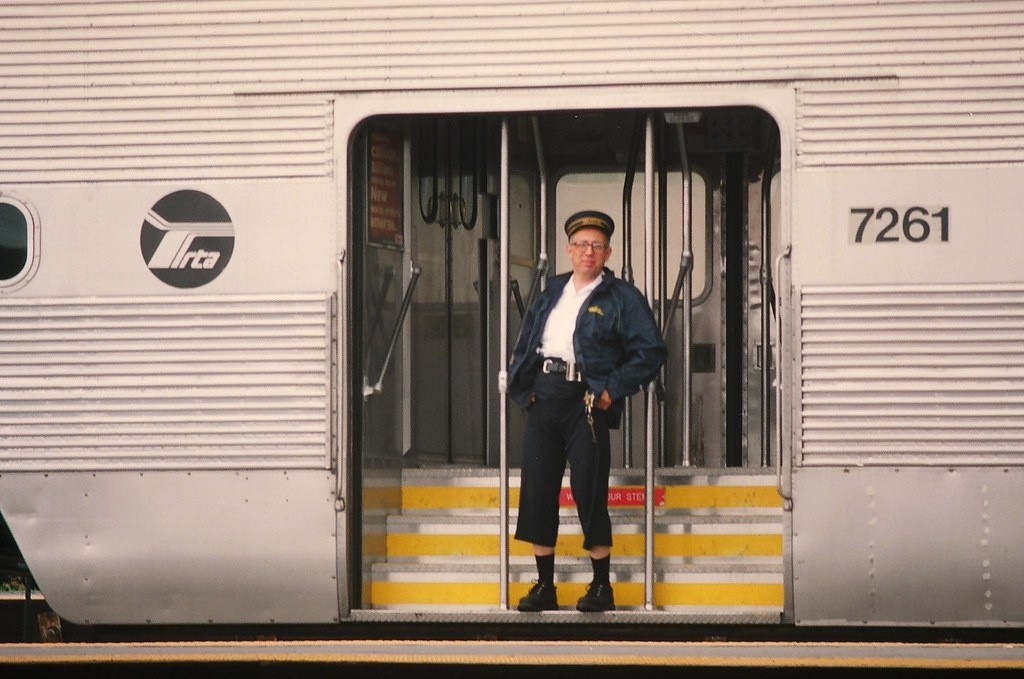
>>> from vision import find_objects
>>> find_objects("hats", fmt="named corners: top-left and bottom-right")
top-left (564, 210), bottom-right (615, 241)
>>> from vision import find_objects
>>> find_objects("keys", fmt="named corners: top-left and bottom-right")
top-left (583, 389), bottom-right (598, 443)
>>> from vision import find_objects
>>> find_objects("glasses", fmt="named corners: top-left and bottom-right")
top-left (570, 240), bottom-right (607, 254)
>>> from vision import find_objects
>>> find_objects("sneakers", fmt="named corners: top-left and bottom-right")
top-left (517, 581), bottom-right (560, 612)
top-left (576, 579), bottom-right (616, 611)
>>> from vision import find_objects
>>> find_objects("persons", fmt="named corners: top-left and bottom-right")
top-left (506, 211), bottom-right (666, 610)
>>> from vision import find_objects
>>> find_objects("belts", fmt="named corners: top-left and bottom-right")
top-left (538, 359), bottom-right (581, 379)
